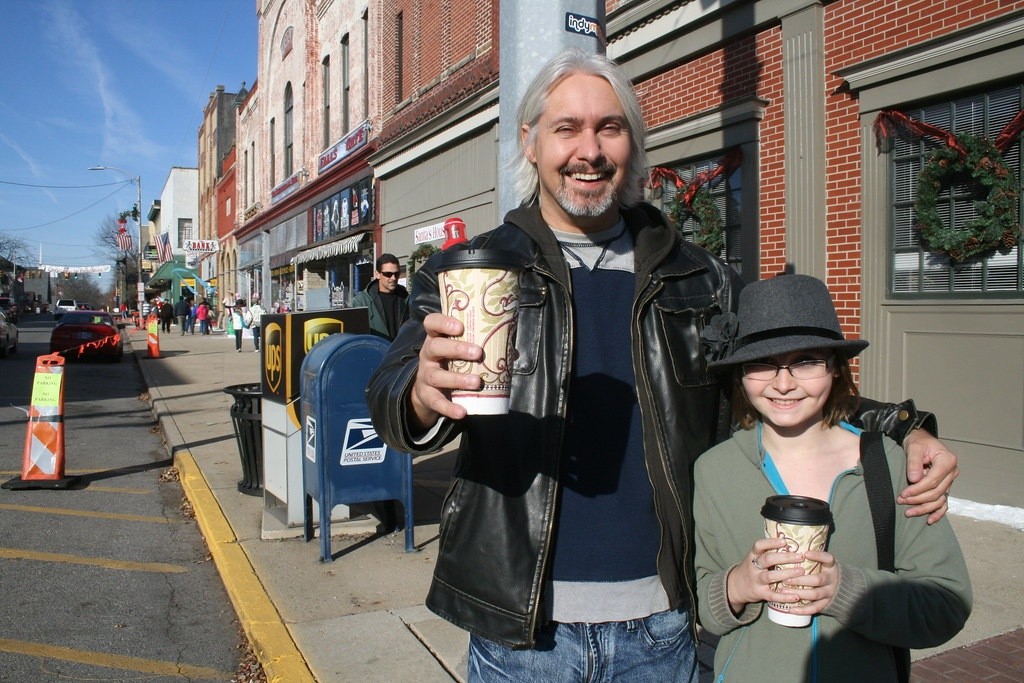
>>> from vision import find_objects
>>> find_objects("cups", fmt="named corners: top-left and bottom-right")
top-left (760, 495), bottom-right (833, 627)
top-left (436, 247), bottom-right (524, 416)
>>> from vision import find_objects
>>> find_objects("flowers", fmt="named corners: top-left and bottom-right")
top-left (910, 130), bottom-right (1020, 263)
top-left (663, 186), bottom-right (725, 258)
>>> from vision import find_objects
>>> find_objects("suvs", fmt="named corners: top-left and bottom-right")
top-left (54, 299), bottom-right (80, 320)
top-left (0, 296), bottom-right (19, 325)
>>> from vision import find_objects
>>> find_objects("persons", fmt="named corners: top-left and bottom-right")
top-left (150, 295), bottom-right (267, 353)
top-left (694, 275), bottom-right (974, 682)
top-left (120, 303), bottom-right (127, 320)
top-left (366, 46), bottom-right (959, 683)
top-left (351, 253), bottom-right (412, 343)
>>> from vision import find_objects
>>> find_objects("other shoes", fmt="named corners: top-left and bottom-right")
top-left (238, 349), bottom-right (241, 352)
top-left (255, 350), bottom-right (259, 353)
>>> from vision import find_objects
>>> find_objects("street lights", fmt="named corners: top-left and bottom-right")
top-left (88, 165), bottom-right (146, 330)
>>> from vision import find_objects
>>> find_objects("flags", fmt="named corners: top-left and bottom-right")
top-left (115, 233), bottom-right (132, 251)
top-left (153, 232), bottom-right (174, 262)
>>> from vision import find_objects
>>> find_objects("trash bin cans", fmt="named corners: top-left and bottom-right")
top-left (223, 382), bottom-right (263, 497)
top-left (300, 333), bottom-right (419, 564)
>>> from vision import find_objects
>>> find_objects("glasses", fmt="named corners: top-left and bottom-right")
top-left (378, 271), bottom-right (401, 278)
top-left (740, 358), bottom-right (832, 381)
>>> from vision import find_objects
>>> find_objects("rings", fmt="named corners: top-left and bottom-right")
top-left (943, 492), bottom-right (949, 499)
top-left (752, 556), bottom-right (764, 569)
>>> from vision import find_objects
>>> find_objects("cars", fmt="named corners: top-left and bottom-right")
top-left (49, 309), bottom-right (125, 363)
top-left (108, 312), bottom-right (126, 329)
top-left (0, 307), bottom-right (19, 358)
top-left (77, 302), bottom-right (92, 311)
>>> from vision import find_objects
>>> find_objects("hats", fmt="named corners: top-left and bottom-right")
top-left (698, 273), bottom-right (868, 371)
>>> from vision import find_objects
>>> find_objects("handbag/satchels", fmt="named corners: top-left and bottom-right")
top-left (228, 320), bottom-right (234, 335)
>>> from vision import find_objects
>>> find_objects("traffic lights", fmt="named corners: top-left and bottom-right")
top-left (74, 273), bottom-right (78, 280)
top-left (98, 272), bottom-right (102, 278)
